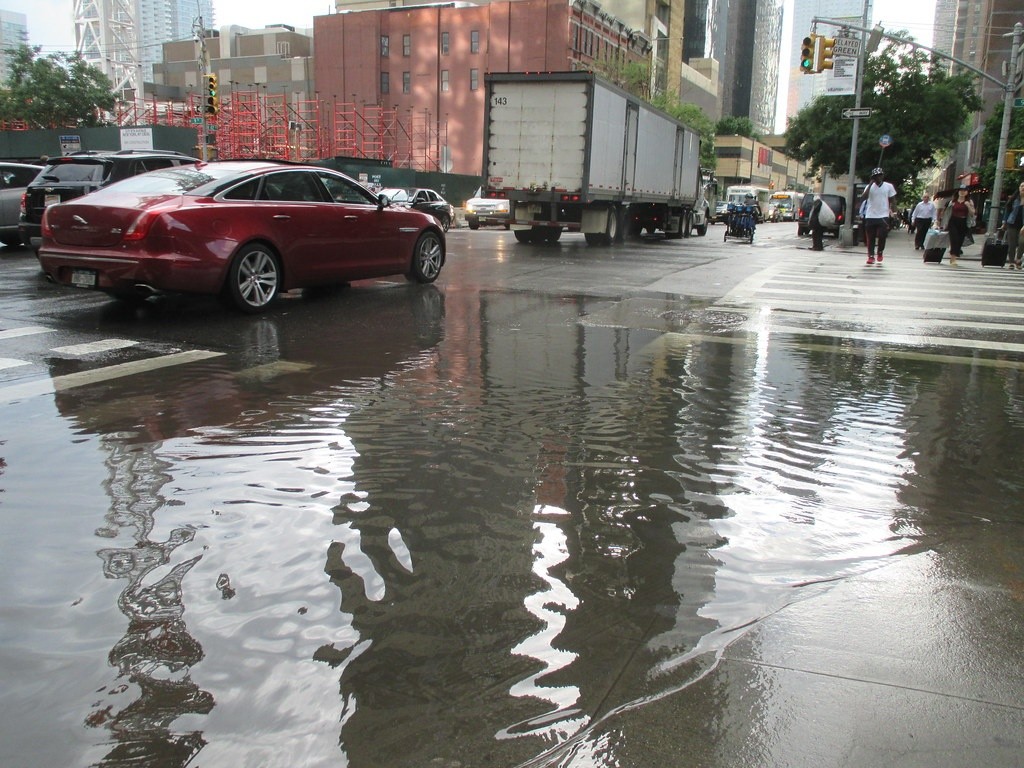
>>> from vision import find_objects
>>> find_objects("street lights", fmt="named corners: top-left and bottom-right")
top-left (193, 32), bottom-right (207, 162)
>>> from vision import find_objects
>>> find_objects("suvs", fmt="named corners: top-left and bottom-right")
top-left (798, 193), bottom-right (846, 238)
top-left (465, 185), bottom-right (534, 230)
top-left (18, 149), bottom-right (202, 259)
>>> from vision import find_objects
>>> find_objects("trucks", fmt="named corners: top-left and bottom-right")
top-left (481, 70), bottom-right (704, 246)
top-left (822, 166), bottom-right (864, 196)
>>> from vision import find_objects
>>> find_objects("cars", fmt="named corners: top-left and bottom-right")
top-left (0, 162), bottom-right (45, 245)
top-left (375, 187), bottom-right (455, 233)
top-left (38, 161), bottom-right (446, 314)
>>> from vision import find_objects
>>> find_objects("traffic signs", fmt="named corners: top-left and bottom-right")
top-left (841, 109), bottom-right (872, 119)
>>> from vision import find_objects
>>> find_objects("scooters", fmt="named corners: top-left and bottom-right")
top-left (724, 202), bottom-right (760, 244)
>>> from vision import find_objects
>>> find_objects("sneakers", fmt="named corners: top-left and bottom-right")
top-left (867, 257), bottom-right (875, 263)
top-left (877, 250), bottom-right (883, 262)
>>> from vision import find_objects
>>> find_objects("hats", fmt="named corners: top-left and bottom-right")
top-left (959, 185), bottom-right (968, 190)
top-left (870, 168), bottom-right (883, 177)
top-left (813, 195), bottom-right (820, 201)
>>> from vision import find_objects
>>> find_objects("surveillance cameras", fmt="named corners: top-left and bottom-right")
top-left (193, 36), bottom-right (201, 43)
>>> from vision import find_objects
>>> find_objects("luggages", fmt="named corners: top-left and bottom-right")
top-left (982, 228), bottom-right (1009, 268)
top-left (923, 229), bottom-right (949, 265)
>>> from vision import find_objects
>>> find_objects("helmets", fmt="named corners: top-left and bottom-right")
top-left (745, 193), bottom-right (753, 198)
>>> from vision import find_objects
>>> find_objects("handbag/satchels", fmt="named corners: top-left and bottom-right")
top-left (968, 202), bottom-right (977, 228)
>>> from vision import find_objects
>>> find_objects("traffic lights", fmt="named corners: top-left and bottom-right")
top-left (800, 34), bottom-right (816, 69)
top-left (818, 36), bottom-right (836, 72)
top-left (208, 75), bottom-right (218, 114)
top-left (1018, 153), bottom-right (1024, 167)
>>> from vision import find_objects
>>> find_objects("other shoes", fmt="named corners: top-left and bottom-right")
top-left (809, 246), bottom-right (823, 251)
top-left (1016, 264), bottom-right (1022, 269)
top-left (1010, 262), bottom-right (1014, 269)
top-left (916, 245), bottom-right (924, 250)
top-left (950, 258), bottom-right (957, 268)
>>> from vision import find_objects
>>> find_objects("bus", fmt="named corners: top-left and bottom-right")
top-left (693, 176), bottom-right (803, 224)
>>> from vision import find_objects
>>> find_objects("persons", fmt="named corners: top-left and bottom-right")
top-left (744, 194), bottom-right (761, 220)
top-left (859, 180), bottom-right (1024, 269)
top-left (40, 154), bottom-right (49, 161)
top-left (808, 193), bottom-right (828, 251)
top-left (861, 168), bottom-right (897, 265)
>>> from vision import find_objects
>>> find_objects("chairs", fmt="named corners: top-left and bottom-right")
top-left (267, 183), bottom-right (281, 201)
